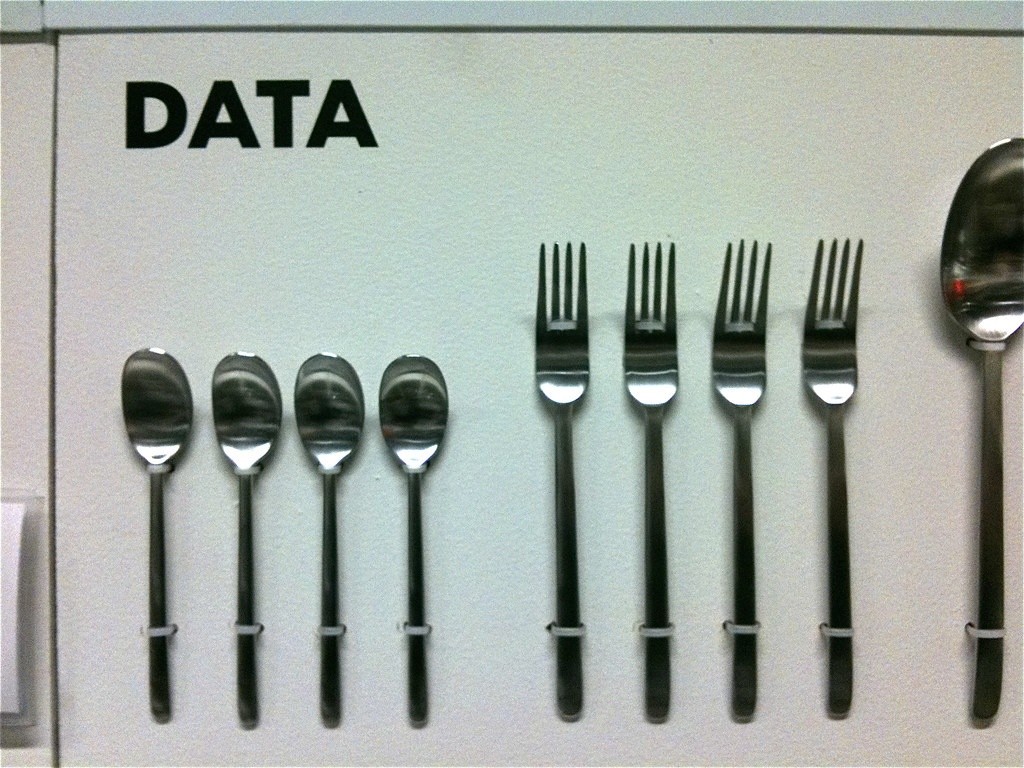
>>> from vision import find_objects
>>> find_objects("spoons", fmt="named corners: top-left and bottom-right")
top-left (124, 348), bottom-right (194, 721)
top-left (296, 352), bottom-right (364, 727)
top-left (939, 132), bottom-right (1024, 724)
top-left (214, 351), bottom-right (284, 727)
top-left (380, 354), bottom-right (448, 726)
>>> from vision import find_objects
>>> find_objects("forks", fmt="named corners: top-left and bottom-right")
top-left (712, 241), bottom-right (772, 721)
top-left (535, 241), bottom-right (590, 721)
top-left (804, 239), bottom-right (865, 719)
top-left (625, 241), bottom-right (679, 724)
